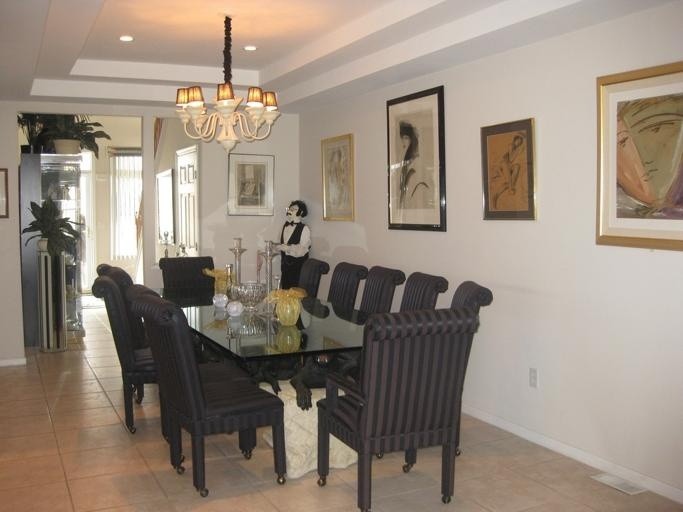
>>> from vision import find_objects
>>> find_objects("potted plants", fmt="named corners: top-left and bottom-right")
top-left (21, 196), bottom-right (85, 264)
top-left (17, 112), bottom-right (112, 159)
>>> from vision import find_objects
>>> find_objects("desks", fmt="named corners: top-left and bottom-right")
top-left (181, 300), bottom-right (373, 461)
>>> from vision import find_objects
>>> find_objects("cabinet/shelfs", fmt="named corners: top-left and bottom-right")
top-left (20, 153), bottom-right (85, 347)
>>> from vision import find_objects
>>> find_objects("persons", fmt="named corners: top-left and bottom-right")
top-left (618, 95), bottom-right (679, 218)
top-left (487, 132), bottom-right (524, 210)
top-left (395, 119), bottom-right (435, 210)
top-left (269, 199), bottom-right (312, 291)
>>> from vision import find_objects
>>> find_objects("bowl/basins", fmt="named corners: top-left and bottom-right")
top-left (224, 313), bottom-right (267, 338)
top-left (227, 285), bottom-right (266, 311)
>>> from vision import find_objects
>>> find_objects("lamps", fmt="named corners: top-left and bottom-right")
top-left (174, 16), bottom-right (281, 156)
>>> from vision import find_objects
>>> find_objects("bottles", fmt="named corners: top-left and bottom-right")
top-left (272, 279), bottom-right (282, 320)
top-left (223, 264), bottom-right (233, 299)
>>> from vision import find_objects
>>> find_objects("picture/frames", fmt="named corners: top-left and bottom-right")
top-left (321, 134), bottom-right (354, 222)
top-left (480, 118), bottom-right (537, 221)
top-left (386, 85), bottom-right (446, 232)
top-left (0, 169), bottom-right (8, 218)
top-left (227, 153), bottom-right (274, 217)
top-left (597, 60), bottom-right (683, 251)
top-left (155, 169), bottom-right (175, 246)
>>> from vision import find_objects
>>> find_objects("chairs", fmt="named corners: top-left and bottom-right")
top-left (126, 291), bottom-right (288, 498)
top-left (127, 284), bottom-right (261, 455)
top-left (96, 265), bottom-right (145, 404)
top-left (275, 254), bottom-right (332, 298)
top-left (157, 256), bottom-right (217, 307)
top-left (400, 271), bottom-right (449, 312)
top-left (450, 280), bottom-right (494, 309)
top-left (93, 275), bottom-right (159, 436)
top-left (326, 262), bottom-right (369, 309)
top-left (360, 266), bottom-right (407, 313)
top-left (314, 305), bottom-right (481, 512)
